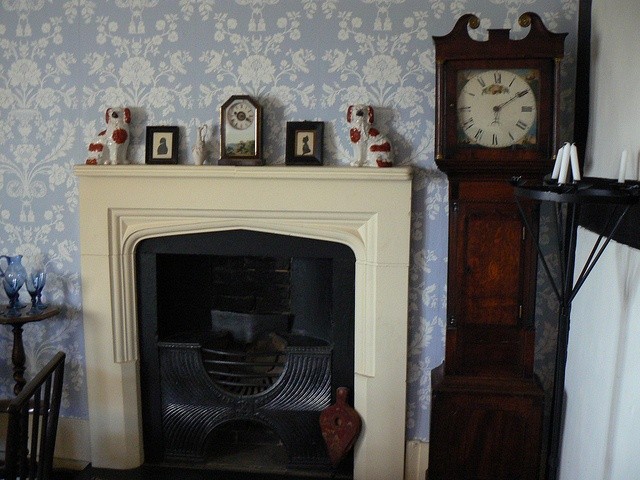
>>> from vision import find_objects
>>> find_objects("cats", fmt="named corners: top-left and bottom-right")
top-left (301, 136), bottom-right (311, 155)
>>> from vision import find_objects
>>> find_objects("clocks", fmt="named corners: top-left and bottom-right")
top-left (218, 94), bottom-right (265, 166)
top-left (432, 12), bottom-right (568, 167)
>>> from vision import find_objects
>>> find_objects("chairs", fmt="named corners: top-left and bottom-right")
top-left (0, 351), bottom-right (65, 479)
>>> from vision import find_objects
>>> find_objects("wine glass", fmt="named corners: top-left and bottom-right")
top-left (24, 275), bottom-right (44, 316)
top-left (30, 268), bottom-right (48, 310)
top-left (2, 277), bottom-right (22, 317)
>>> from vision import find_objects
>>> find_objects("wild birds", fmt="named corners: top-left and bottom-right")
top-left (157, 137), bottom-right (168, 155)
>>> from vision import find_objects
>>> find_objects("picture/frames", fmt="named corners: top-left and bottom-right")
top-left (286, 121), bottom-right (323, 166)
top-left (146, 126), bottom-right (178, 165)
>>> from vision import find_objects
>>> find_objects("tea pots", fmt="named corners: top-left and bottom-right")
top-left (0, 252), bottom-right (28, 311)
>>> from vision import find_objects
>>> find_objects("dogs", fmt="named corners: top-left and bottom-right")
top-left (85, 107), bottom-right (130, 165)
top-left (347, 104), bottom-right (395, 167)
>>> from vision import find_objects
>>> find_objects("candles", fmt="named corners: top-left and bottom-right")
top-left (619, 150), bottom-right (628, 183)
top-left (559, 143), bottom-right (571, 183)
top-left (552, 147), bottom-right (564, 180)
top-left (571, 144), bottom-right (581, 180)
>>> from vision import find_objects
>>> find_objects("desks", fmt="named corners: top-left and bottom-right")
top-left (0, 304), bottom-right (62, 412)
